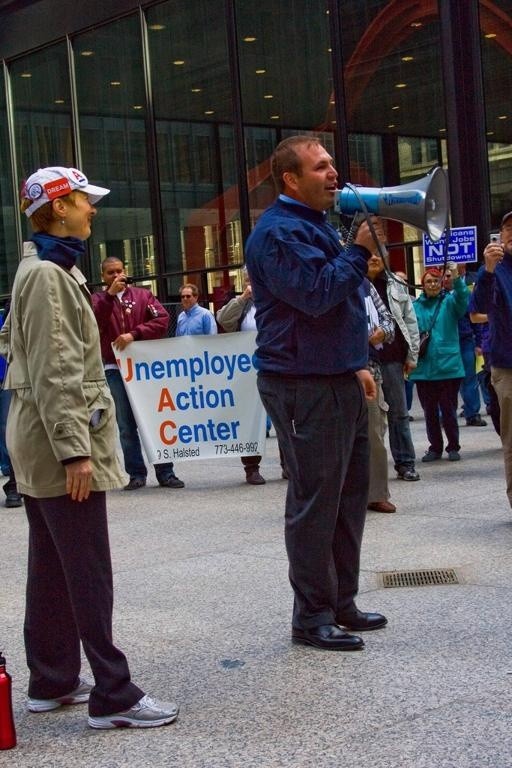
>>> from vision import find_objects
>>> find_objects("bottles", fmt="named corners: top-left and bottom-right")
top-left (0, 651), bottom-right (18, 751)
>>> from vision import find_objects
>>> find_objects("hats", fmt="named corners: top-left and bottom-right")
top-left (500, 211), bottom-right (512, 226)
top-left (21, 165), bottom-right (110, 219)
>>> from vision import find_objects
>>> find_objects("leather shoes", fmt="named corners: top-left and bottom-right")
top-left (246, 471), bottom-right (265, 484)
top-left (291, 625), bottom-right (364, 651)
top-left (6, 488), bottom-right (23, 507)
top-left (335, 601), bottom-right (388, 631)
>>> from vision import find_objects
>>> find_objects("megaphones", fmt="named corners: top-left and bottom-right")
top-left (332, 165), bottom-right (447, 243)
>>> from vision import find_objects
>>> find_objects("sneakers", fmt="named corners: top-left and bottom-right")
top-left (369, 414), bottom-right (487, 512)
top-left (29, 677), bottom-right (94, 712)
top-left (160, 474), bottom-right (184, 488)
top-left (88, 694), bottom-right (180, 729)
top-left (124, 475), bottom-right (146, 490)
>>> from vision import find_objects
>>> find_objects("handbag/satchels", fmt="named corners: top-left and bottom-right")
top-left (419, 331), bottom-right (430, 357)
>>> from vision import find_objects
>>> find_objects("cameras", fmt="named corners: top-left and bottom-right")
top-left (490, 234), bottom-right (502, 244)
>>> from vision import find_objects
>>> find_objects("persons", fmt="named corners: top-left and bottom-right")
top-left (244, 134), bottom-right (387, 651)
top-left (0, 164), bottom-right (179, 729)
